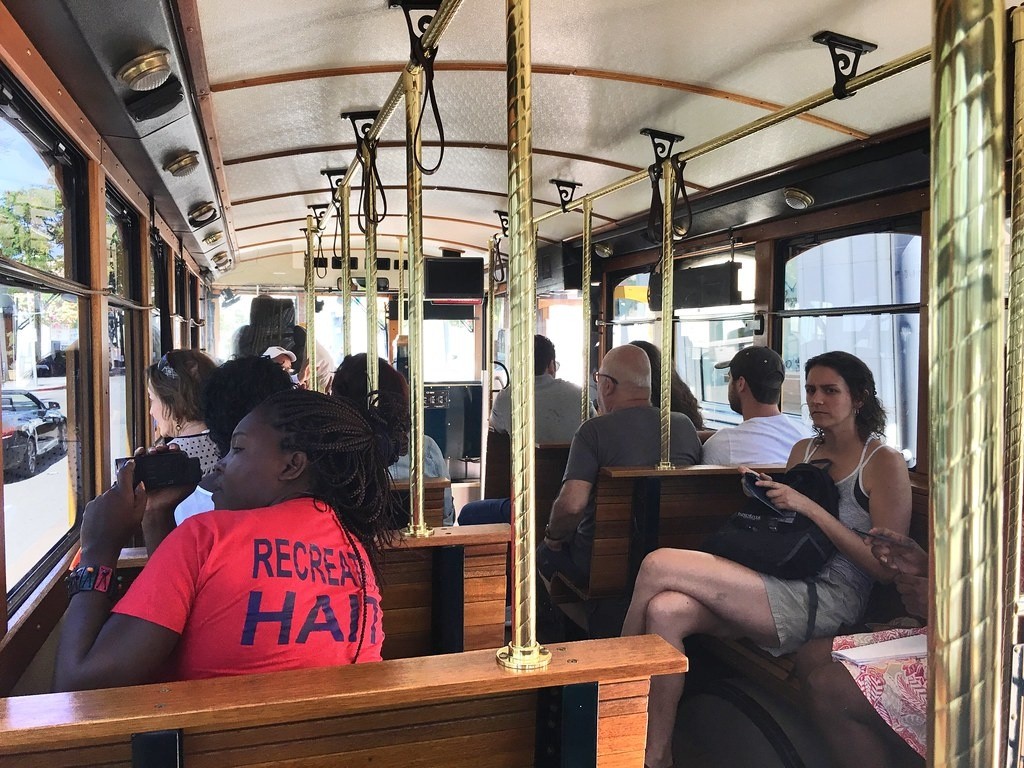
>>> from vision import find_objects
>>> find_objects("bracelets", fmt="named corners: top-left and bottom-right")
top-left (68, 566), bottom-right (129, 602)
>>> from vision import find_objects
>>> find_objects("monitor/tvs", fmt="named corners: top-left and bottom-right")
top-left (424, 257), bottom-right (484, 299)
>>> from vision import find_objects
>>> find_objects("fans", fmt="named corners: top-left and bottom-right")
top-left (207, 287), bottom-right (241, 309)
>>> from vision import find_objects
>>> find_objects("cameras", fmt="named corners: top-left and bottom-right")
top-left (114, 450), bottom-right (203, 491)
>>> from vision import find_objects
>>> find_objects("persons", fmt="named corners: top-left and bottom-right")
top-left (51, 388), bottom-right (411, 694)
top-left (618, 351), bottom-right (914, 767)
top-left (51, 333), bottom-right (822, 694)
top-left (863, 527), bottom-right (930, 621)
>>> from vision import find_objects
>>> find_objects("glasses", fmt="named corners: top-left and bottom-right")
top-left (593, 371), bottom-right (618, 384)
top-left (157, 351), bottom-right (179, 379)
top-left (548, 360), bottom-right (560, 372)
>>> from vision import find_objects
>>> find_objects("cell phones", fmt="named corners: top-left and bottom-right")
top-left (745, 471), bottom-right (766, 487)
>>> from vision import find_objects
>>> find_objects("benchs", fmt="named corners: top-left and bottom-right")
top-left (0, 427), bottom-right (935, 768)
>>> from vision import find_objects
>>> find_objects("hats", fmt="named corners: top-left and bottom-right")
top-left (261, 346), bottom-right (297, 363)
top-left (715, 345), bottom-right (786, 389)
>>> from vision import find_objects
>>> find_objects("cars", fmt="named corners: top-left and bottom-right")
top-left (1, 390), bottom-right (68, 477)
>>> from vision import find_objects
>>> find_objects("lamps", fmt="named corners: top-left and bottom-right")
top-left (672, 223), bottom-right (687, 241)
top-left (188, 201), bottom-right (215, 222)
top-left (594, 243), bottom-right (614, 260)
top-left (164, 151), bottom-right (200, 178)
top-left (201, 230), bottom-right (224, 246)
top-left (212, 250), bottom-right (231, 270)
top-left (783, 187), bottom-right (815, 211)
top-left (114, 49), bottom-right (174, 93)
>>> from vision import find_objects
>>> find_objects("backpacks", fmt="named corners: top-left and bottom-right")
top-left (698, 458), bottom-right (842, 578)
